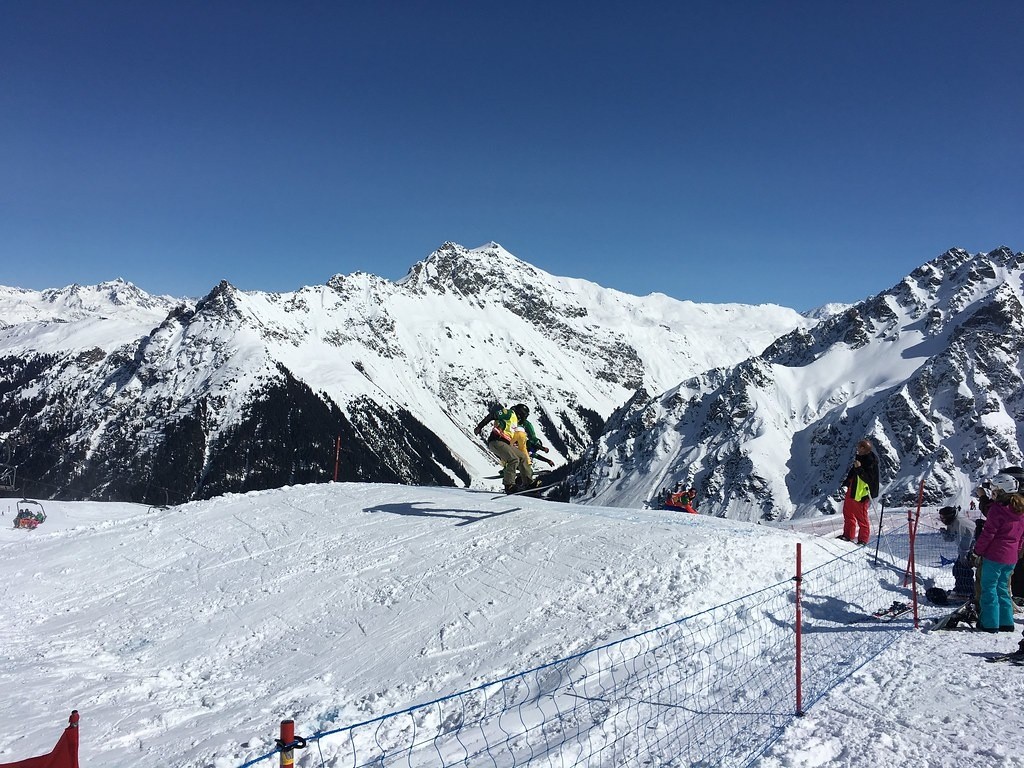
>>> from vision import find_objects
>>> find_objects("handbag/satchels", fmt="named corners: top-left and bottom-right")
top-left (490, 424), bottom-right (511, 445)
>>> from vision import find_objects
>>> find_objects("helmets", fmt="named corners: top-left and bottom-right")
top-left (989, 472), bottom-right (1019, 493)
top-left (510, 404), bottom-right (530, 426)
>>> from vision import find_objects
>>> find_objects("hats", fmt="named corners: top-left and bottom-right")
top-left (860, 440), bottom-right (872, 449)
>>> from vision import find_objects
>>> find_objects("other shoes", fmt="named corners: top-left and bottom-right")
top-left (975, 622), bottom-right (999, 633)
top-left (999, 625), bottom-right (1015, 632)
top-left (836, 535), bottom-right (851, 541)
top-left (505, 484), bottom-right (525, 495)
top-left (521, 479), bottom-right (543, 490)
top-left (856, 540), bottom-right (866, 546)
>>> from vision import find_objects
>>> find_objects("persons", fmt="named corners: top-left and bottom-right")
top-left (665, 487), bottom-right (698, 514)
top-left (939, 466), bottom-right (1024, 634)
top-left (835, 439), bottom-right (880, 543)
top-left (13, 508), bottom-right (43, 528)
top-left (473, 403), bottom-right (554, 496)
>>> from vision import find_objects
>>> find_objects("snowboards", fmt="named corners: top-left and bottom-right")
top-left (491, 482), bottom-right (560, 502)
top-left (483, 469), bottom-right (551, 480)
